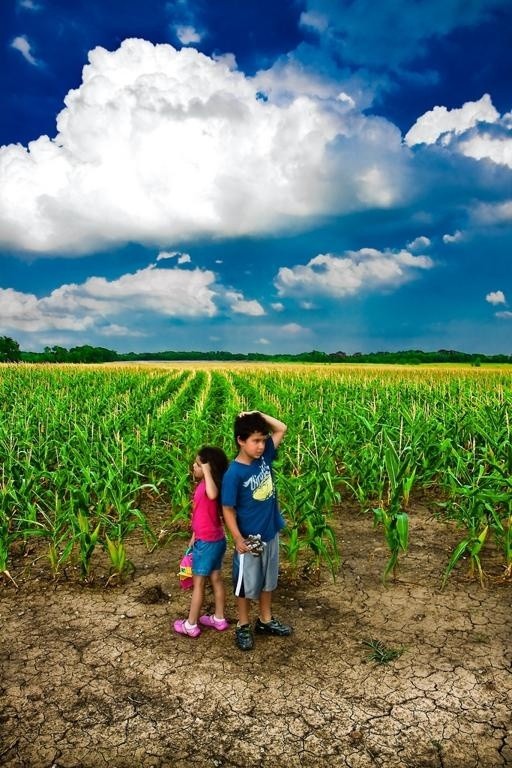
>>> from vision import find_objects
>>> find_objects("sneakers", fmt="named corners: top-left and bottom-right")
top-left (236, 621), bottom-right (254, 650)
top-left (256, 618), bottom-right (291, 637)
top-left (199, 614), bottom-right (229, 631)
top-left (174, 619), bottom-right (202, 638)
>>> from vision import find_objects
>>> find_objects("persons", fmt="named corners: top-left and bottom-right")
top-left (173, 446), bottom-right (231, 637)
top-left (219, 408), bottom-right (292, 650)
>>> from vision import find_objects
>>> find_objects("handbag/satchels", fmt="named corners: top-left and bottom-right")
top-left (178, 547), bottom-right (194, 590)
